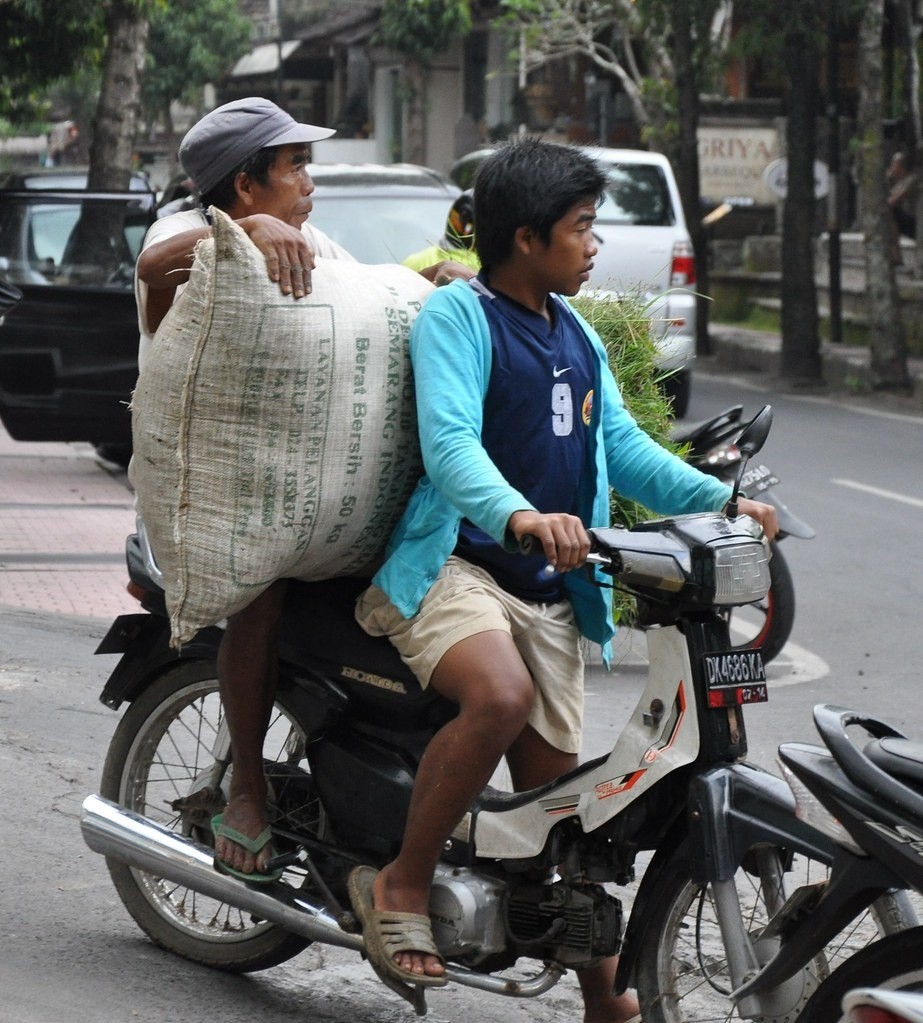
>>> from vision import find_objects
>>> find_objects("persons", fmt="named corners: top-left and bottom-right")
top-left (352, 139), bottom-right (777, 1022)
top-left (403, 189), bottom-right (482, 275)
top-left (135, 95), bottom-right (481, 881)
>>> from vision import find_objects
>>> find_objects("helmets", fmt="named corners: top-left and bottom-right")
top-left (443, 186), bottom-right (475, 249)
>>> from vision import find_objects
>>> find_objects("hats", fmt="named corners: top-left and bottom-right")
top-left (178, 96), bottom-right (338, 196)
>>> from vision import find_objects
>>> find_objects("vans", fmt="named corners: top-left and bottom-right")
top-left (0, 140), bottom-right (701, 463)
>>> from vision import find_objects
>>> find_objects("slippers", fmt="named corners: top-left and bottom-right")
top-left (345, 864), bottom-right (451, 987)
top-left (210, 811), bottom-right (285, 884)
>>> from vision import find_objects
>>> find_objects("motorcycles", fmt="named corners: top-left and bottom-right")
top-left (74, 403), bottom-right (923, 1023)
